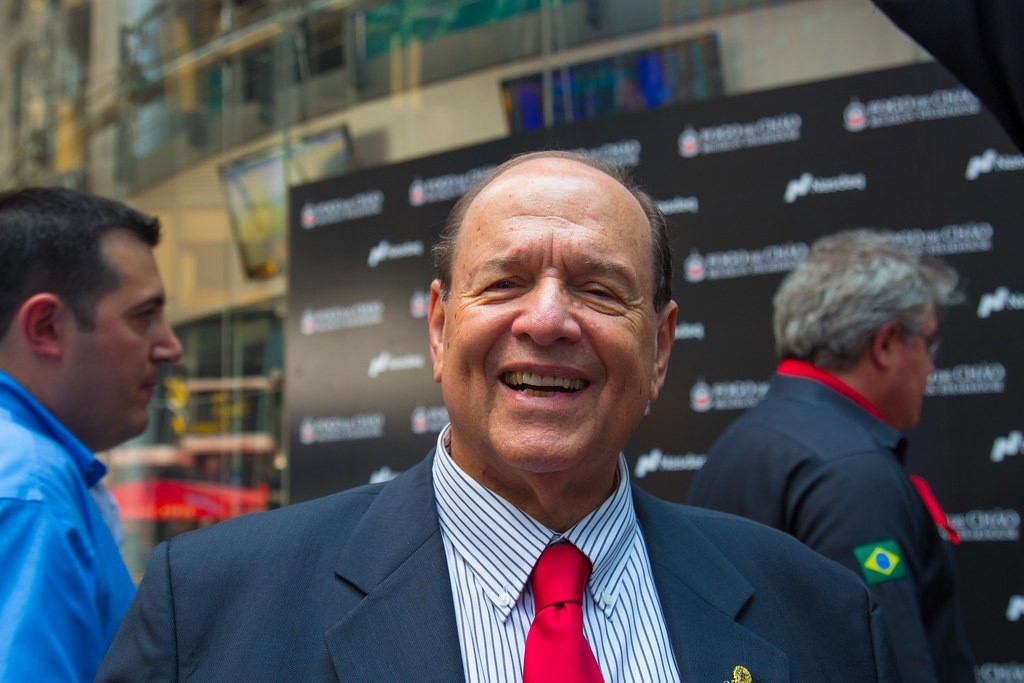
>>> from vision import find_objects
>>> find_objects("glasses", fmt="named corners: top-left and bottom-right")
top-left (898, 323), bottom-right (946, 353)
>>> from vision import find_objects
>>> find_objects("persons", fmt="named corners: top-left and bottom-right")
top-left (685, 228), bottom-right (976, 682)
top-left (1, 182), bottom-right (185, 683)
top-left (90, 146), bottom-right (900, 683)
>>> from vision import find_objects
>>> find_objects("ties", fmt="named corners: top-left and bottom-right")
top-left (522, 548), bottom-right (607, 683)
